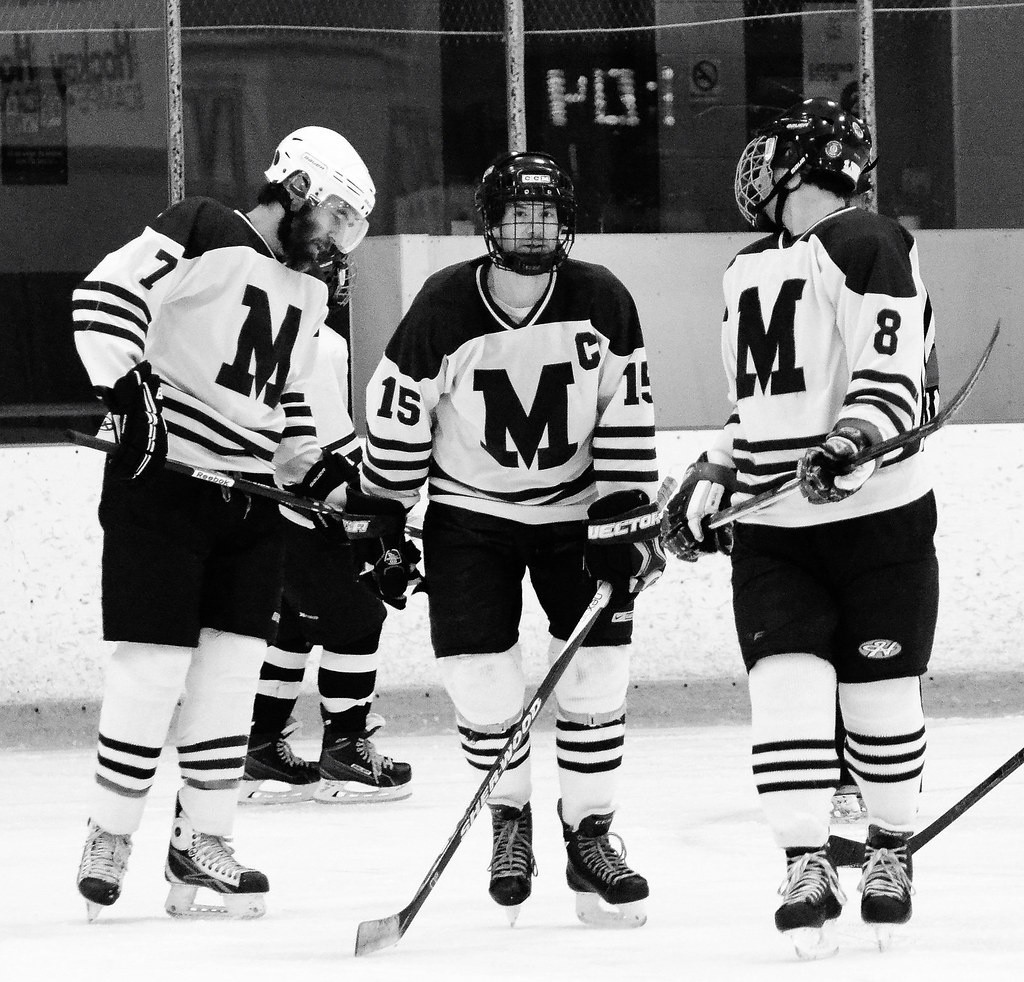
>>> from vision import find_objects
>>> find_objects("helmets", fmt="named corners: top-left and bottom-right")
top-left (264, 125), bottom-right (377, 218)
top-left (474, 152), bottom-right (577, 275)
top-left (734, 97), bottom-right (874, 227)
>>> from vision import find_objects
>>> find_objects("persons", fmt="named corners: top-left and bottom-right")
top-left (70, 124), bottom-right (415, 906)
top-left (656, 94), bottom-right (940, 933)
top-left (359, 152), bottom-right (664, 909)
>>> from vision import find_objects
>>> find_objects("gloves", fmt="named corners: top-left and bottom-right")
top-left (92, 363), bottom-right (166, 482)
top-left (796, 419), bottom-right (884, 504)
top-left (282, 451), bottom-right (360, 550)
top-left (660, 450), bottom-right (737, 563)
top-left (342, 499), bottom-right (407, 601)
top-left (583, 489), bottom-right (667, 610)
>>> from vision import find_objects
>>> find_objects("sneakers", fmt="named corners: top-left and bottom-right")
top-left (237, 737), bottom-right (321, 804)
top-left (75, 816), bottom-right (134, 924)
top-left (774, 843), bottom-right (849, 958)
top-left (484, 800), bottom-right (538, 928)
top-left (555, 796), bottom-right (650, 930)
top-left (313, 713), bottom-right (413, 804)
top-left (859, 824), bottom-right (914, 953)
top-left (163, 789), bottom-right (270, 923)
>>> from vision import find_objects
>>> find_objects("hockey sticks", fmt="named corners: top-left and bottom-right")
top-left (353, 475), bottom-right (678, 961)
top-left (709, 314), bottom-right (1006, 530)
top-left (67, 426), bottom-right (426, 544)
top-left (824, 746), bottom-right (1024, 867)
